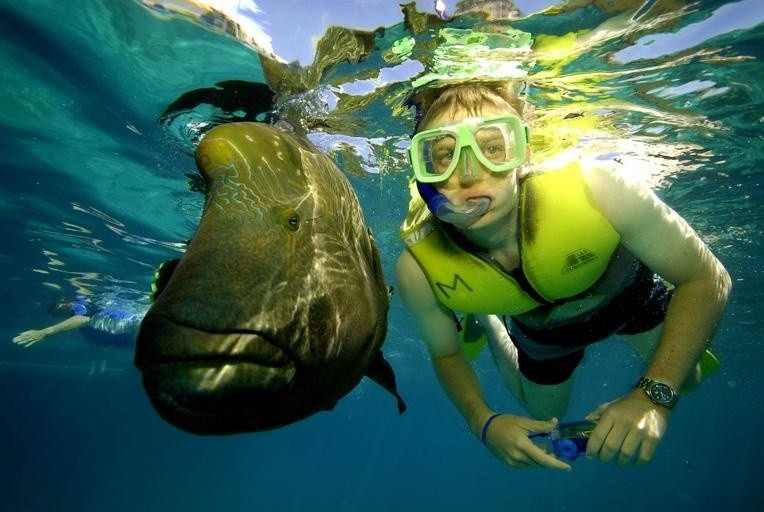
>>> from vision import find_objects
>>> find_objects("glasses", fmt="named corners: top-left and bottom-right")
top-left (404, 114), bottom-right (530, 184)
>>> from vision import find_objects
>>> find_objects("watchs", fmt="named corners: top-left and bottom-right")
top-left (635, 375), bottom-right (680, 410)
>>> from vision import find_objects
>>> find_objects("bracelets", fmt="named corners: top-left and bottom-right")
top-left (481, 412), bottom-right (504, 448)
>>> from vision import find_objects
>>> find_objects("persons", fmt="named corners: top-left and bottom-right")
top-left (9, 295), bottom-right (150, 348)
top-left (394, 80), bottom-right (732, 472)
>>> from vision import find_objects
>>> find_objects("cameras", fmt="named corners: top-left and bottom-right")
top-left (549, 419), bottom-right (600, 460)
